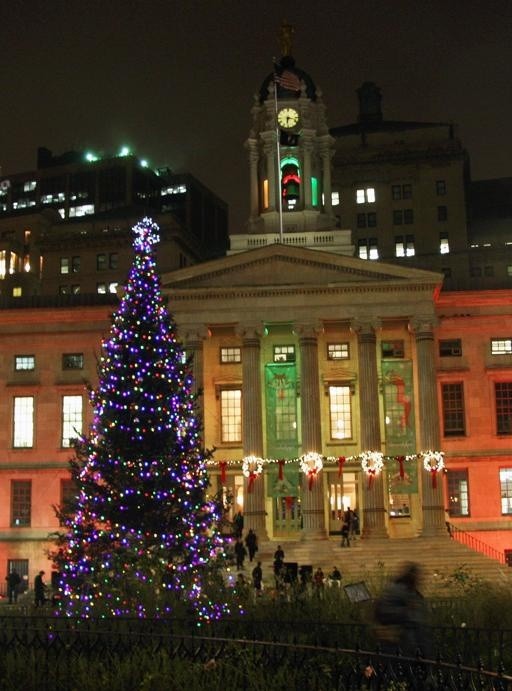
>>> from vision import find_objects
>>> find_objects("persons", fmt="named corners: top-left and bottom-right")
top-left (401, 504), bottom-right (409, 514)
top-left (346, 512), bottom-right (358, 542)
top-left (370, 561), bottom-right (426, 653)
top-left (273, 557), bottom-right (341, 602)
top-left (252, 562), bottom-right (262, 589)
top-left (344, 506), bottom-right (359, 526)
top-left (235, 574), bottom-right (249, 589)
top-left (274, 546), bottom-right (284, 562)
top-left (233, 511), bottom-right (258, 571)
top-left (11, 569), bottom-right (21, 594)
top-left (341, 520), bottom-right (350, 549)
top-left (444, 509), bottom-right (453, 537)
top-left (5, 571), bottom-right (15, 605)
top-left (34, 571), bottom-right (49, 607)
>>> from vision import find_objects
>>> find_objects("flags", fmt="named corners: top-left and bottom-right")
top-left (273, 64), bottom-right (303, 92)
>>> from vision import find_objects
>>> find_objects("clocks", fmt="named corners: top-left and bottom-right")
top-left (276, 107), bottom-right (300, 130)
